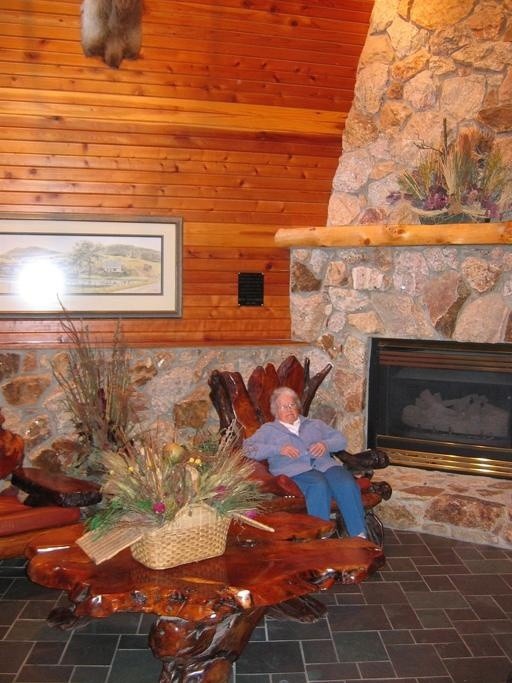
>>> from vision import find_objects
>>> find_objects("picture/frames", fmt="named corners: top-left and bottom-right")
top-left (0, 211), bottom-right (183, 319)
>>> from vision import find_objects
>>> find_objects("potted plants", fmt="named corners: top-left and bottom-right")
top-left (395, 118), bottom-right (509, 224)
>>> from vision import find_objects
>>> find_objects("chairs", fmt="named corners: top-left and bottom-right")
top-left (0, 414), bottom-right (103, 560)
top-left (207, 355), bottom-right (392, 549)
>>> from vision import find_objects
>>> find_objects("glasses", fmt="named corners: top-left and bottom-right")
top-left (277, 402), bottom-right (299, 410)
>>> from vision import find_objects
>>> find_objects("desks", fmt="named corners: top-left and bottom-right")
top-left (24, 511), bottom-right (386, 682)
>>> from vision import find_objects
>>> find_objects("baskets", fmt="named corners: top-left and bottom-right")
top-left (77, 508), bottom-right (276, 570)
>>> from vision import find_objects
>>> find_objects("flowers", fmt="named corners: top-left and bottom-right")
top-left (84, 419), bottom-right (270, 542)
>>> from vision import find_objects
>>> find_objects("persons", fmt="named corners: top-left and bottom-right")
top-left (243, 386), bottom-right (368, 540)
